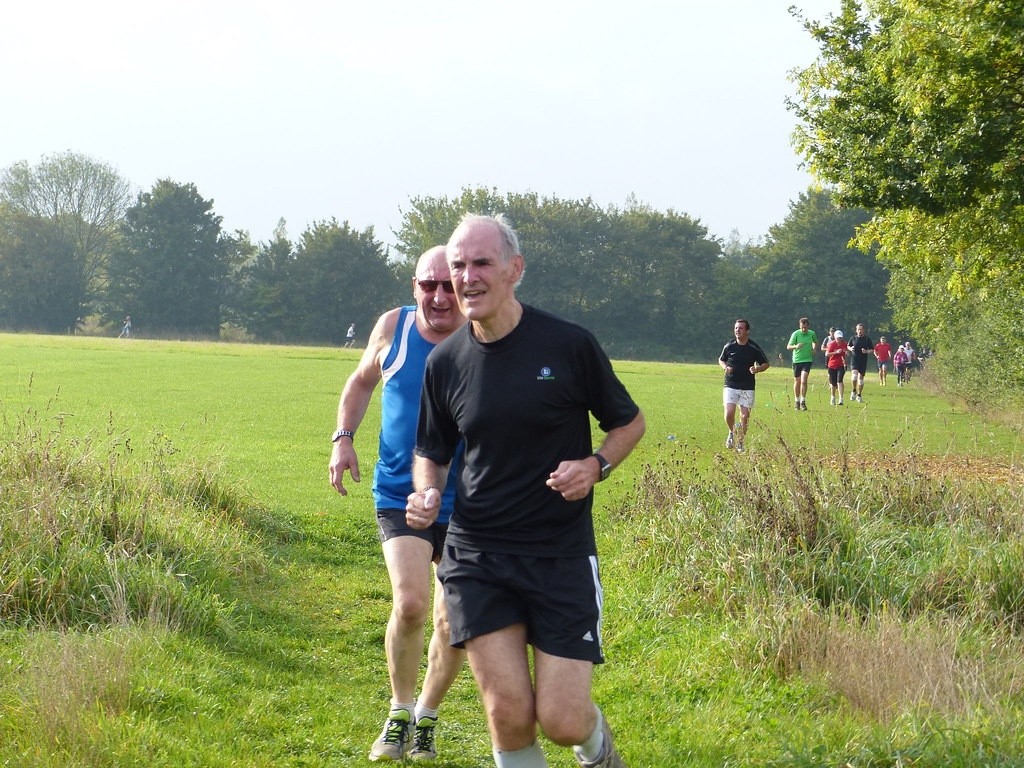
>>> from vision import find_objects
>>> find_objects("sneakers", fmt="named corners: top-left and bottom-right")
top-left (838, 397), bottom-right (844, 406)
top-left (369, 710), bottom-right (418, 763)
top-left (800, 401), bottom-right (807, 411)
top-left (735, 439), bottom-right (745, 454)
top-left (726, 428), bottom-right (737, 449)
top-left (830, 396), bottom-right (836, 405)
top-left (572, 715), bottom-right (626, 768)
top-left (850, 391), bottom-right (857, 401)
top-left (794, 400), bottom-right (801, 411)
top-left (856, 395), bottom-right (862, 403)
top-left (405, 716), bottom-right (441, 765)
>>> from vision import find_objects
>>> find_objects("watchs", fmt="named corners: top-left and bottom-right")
top-left (331, 429), bottom-right (355, 443)
top-left (591, 452), bottom-right (610, 482)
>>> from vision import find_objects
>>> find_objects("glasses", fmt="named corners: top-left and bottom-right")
top-left (415, 279), bottom-right (456, 295)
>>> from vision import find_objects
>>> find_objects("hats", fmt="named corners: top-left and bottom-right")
top-left (834, 330), bottom-right (844, 339)
top-left (898, 345), bottom-right (905, 349)
top-left (829, 327), bottom-right (836, 333)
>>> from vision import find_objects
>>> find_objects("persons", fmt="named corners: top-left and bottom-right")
top-left (718, 318), bottom-right (768, 454)
top-left (821, 328), bottom-right (838, 366)
top-left (903, 341), bottom-right (914, 381)
top-left (329, 244), bottom-right (480, 758)
top-left (825, 330), bottom-right (848, 406)
top-left (893, 345), bottom-right (907, 385)
top-left (919, 348), bottom-right (930, 370)
top-left (847, 323), bottom-right (873, 404)
top-left (787, 318), bottom-right (817, 411)
top-left (874, 335), bottom-right (891, 386)
top-left (407, 213), bottom-right (649, 768)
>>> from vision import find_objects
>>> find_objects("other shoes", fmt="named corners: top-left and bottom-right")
top-left (880, 379), bottom-right (887, 387)
top-left (898, 382), bottom-right (904, 388)
